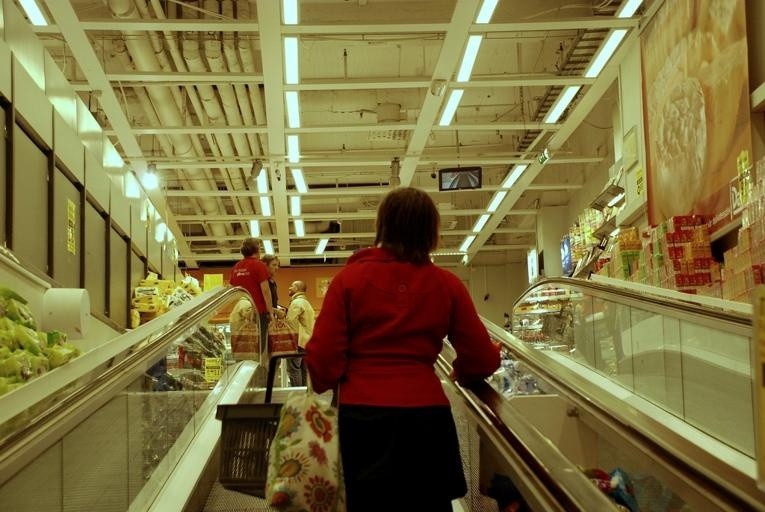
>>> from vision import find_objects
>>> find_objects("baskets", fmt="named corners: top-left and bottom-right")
top-left (215, 351), bottom-right (303, 500)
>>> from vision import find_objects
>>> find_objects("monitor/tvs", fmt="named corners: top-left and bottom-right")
top-left (527, 247), bottom-right (540, 285)
top-left (560, 238), bottom-right (571, 273)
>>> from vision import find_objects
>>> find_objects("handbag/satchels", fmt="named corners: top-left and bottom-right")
top-left (267, 310), bottom-right (298, 356)
top-left (265, 371), bottom-right (347, 512)
top-left (230, 311), bottom-right (258, 359)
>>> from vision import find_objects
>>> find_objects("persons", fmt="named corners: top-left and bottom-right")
top-left (230, 237), bottom-right (274, 353)
top-left (261, 254), bottom-right (280, 321)
top-left (286, 280), bottom-right (316, 350)
top-left (303, 187), bottom-right (501, 512)
